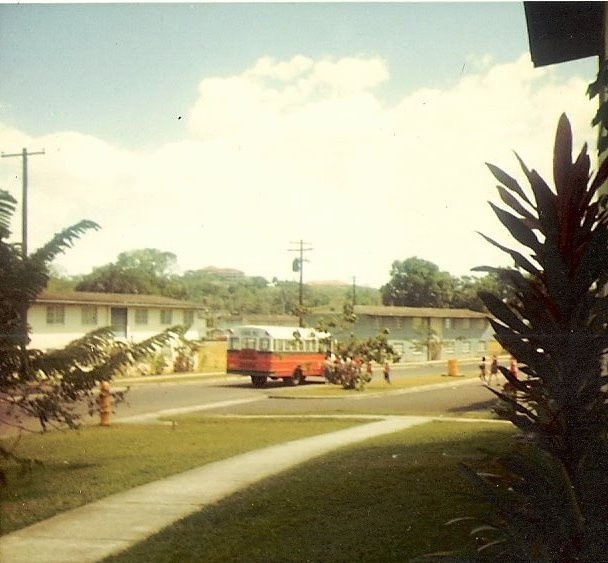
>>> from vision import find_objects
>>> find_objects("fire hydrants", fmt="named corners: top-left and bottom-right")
top-left (97, 378), bottom-right (114, 425)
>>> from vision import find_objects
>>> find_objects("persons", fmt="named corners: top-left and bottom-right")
top-left (479, 357), bottom-right (488, 384)
top-left (491, 355), bottom-right (499, 385)
top-left (324, 350), bottom-right (376, 388)
top-left (382, 361), bottom-right (394, 385)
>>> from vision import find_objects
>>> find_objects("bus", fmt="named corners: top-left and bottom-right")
top-left (226, 325), bottom-right (336, 387)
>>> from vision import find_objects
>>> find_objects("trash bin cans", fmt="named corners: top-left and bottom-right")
top-left (447, 357), bottom-right (459, 376)
